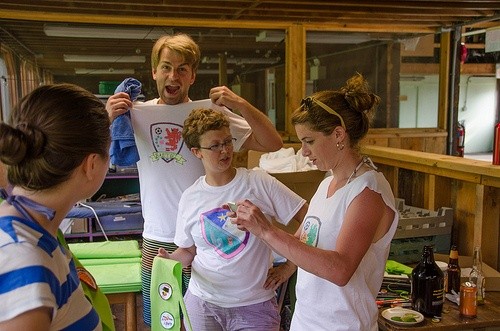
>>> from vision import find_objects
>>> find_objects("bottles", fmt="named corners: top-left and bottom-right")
top-left (448, 245), bottom-right (460, 295)
top-left (412, 245), bottom-right (444, 318)
top-left (469, 246), bottom-right (487, 305)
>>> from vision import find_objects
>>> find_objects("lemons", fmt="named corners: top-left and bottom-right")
top-left (390, 317), bottom-right (417, 323)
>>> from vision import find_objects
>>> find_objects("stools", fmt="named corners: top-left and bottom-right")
top-left (104, 292), bottom-right (137, 331)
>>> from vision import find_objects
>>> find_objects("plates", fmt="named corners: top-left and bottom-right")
top-left (381, 307), bottom-right (424, 326)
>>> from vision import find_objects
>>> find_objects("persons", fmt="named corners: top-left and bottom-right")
top-left (104, 35), bottom-right (283, 331)
top-left (0, 83), bottom-right (112, 331)
top-left (221, 74), bottom-right (398, 331)
top-left (156, 109), bottom-right (309, 331)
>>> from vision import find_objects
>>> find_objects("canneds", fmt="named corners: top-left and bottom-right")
top-left (460, 282), bottom-right (478, 319)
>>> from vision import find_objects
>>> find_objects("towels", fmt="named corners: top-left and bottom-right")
top-left (252, 147), bottom-right (318, 173)
top-left (108, 77), bottom-right (142, 167)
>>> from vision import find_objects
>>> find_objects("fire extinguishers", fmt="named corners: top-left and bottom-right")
top-left (456, 120), bottom-right (465, 157)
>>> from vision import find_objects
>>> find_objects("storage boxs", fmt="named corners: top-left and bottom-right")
top-left (433, 253), bottom-right (500, 292)
top-left (387, 197), bottom-right (453, 264)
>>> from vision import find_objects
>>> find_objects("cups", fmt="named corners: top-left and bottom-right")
top-left (434, 259), bottom-right (448, 291)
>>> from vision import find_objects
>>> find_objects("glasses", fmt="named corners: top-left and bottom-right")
top-left (300, 97), bottom-right (346, 131)
top-left (197, 137), bottom-right (237, 152)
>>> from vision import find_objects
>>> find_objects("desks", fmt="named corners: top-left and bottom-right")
top-left (376, 260), bottom-right (500, 331)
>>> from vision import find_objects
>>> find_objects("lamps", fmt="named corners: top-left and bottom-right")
top-left (43, 23), bottom-right (173, 40)
top-left (201, 56), bottom-right (280, 64)
top-left (63, 54), bottom-right (146, 63)
top-left (307, 57), bottom-right (326, 80)
top-left (196, 69), bottom-right (234, 74)
top-left (74, 68), bottom-right (135, 75)
top-left (255, 31), bottom-right (371, 44)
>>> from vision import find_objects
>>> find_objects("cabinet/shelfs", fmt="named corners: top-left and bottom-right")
top-left (58, 173), bottom-right (145, 243)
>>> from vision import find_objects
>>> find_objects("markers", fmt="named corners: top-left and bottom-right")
top-left (376, 299), bottom-right (412, 307)
